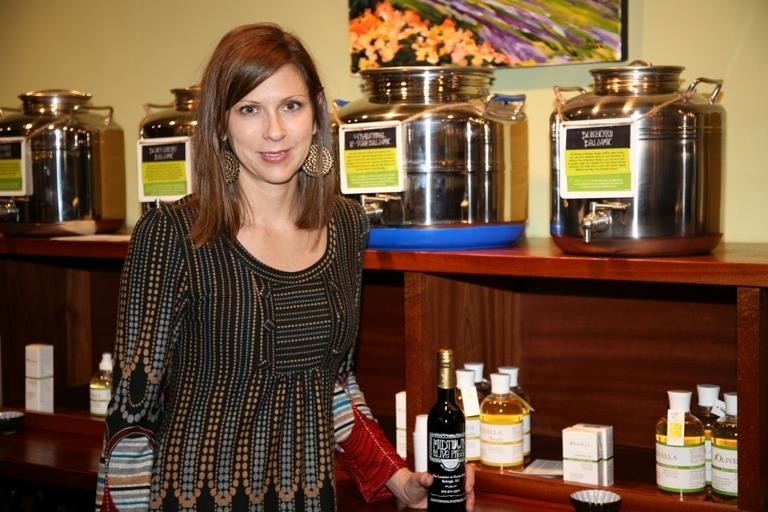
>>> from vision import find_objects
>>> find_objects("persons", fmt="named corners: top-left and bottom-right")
top-left (97, 20), bottom-right (478, 512)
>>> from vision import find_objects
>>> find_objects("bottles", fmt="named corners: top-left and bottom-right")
top-left (428, 349), bottom-right (468, 511)
top-left (654, 383), bottom-right (737, 501)
top-left (456, 362), bottom-right (532, 474)
top-left (90, 349), bottom-right (114, 415)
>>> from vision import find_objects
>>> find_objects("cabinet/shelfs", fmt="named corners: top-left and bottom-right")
top-left (0, 229), bottom-right (768, 512)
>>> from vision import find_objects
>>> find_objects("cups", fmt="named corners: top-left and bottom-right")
top-left (568, 489), bottom-right (622, 511)
